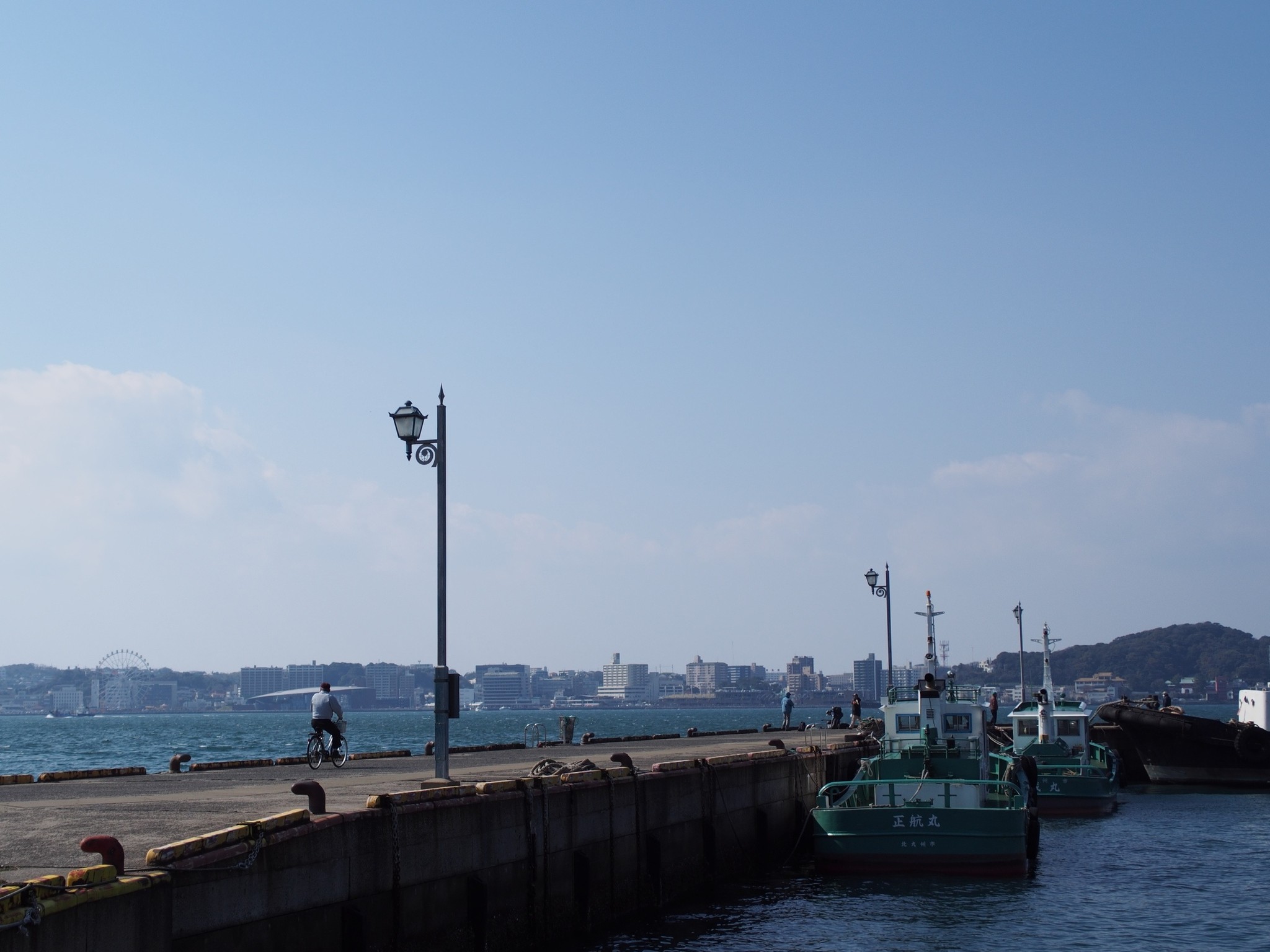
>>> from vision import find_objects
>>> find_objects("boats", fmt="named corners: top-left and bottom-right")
top-left (1087, 696), bottom-right (1270, 786)
top-left (811, 588), bottom-right (1040, 876)
top-left (987, 622), bottom-right (1122, 816)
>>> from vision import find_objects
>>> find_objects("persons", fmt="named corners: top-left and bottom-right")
top-left (781, 692), bottom-right (795, 731)
top-left (848, 693), bottom-right (861, 729)
top-left (1161, 691), bottom-right (1171, 712)
top-left (826, 711), bottom-right (845, 728)
top-left (989, 692), bottom-right (998, 727)
top-left (310, 682), bottom-right (344, 759)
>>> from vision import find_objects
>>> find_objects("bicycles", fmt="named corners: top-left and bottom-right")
top-left (306, 717), bottom-right (350, 769)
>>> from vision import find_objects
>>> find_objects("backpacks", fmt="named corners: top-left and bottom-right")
top-left (832, 706), bottom-right (842, 713)
top-left (797, 722), bottom-right (806, 731)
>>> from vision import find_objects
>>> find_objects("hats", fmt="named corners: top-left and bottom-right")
top-left (1162, 691), bottom-right (1167, 694)
top-left (826, 710), bottom-right (830, 715)
top-left (852, 693), bottom-right (858, 697)
top-left (993, 692), bottom-right (997, 695)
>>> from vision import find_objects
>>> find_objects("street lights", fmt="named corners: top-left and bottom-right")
top-left (864, 561), bottom-right (905, 701)
top-left (1011, 601), bottom-right (1029, 704)
top-left (388, 381), bottom-right (460, 785)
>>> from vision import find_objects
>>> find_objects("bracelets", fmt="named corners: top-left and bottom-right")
top-left (338, 717), bottom-right (343, 720)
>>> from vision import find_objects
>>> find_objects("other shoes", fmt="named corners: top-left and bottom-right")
top-left (781, 727), bottom-right (791, 731)
top-left (846, 727), bottom-right (853, 729)
top-left (332, 750), bottom-right (342, 758)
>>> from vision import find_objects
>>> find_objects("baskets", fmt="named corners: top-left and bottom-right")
top-left (332, 720), bottom-right (347, 733)
